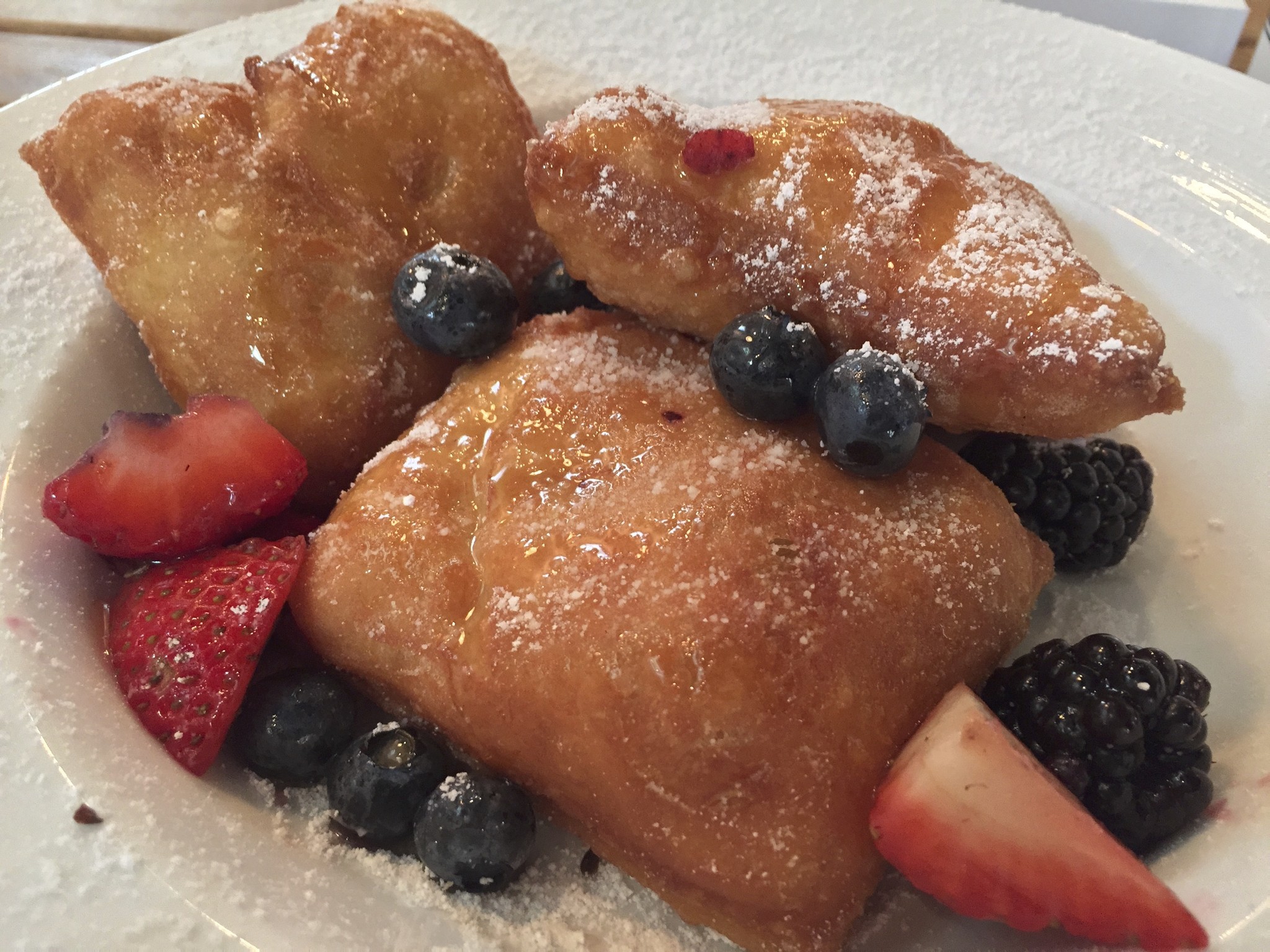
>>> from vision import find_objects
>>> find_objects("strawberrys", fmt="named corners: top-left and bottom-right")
top-left (109, 535), bottom-right (307, 778)
top-left (866, 682), bottom-right (1209, 952)
top-left (40, 396), bottom-right (308, 557)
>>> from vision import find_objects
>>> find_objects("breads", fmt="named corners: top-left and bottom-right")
top-left (518, 85), bottom-right (1183, 445)
top-left (278, 303), bottom-right (1056, 952)
top-left (16, 0), bottom-right (553, 511)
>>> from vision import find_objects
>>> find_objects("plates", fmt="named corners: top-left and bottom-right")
top-left (0, 0), bottom-right (1269, 952)
